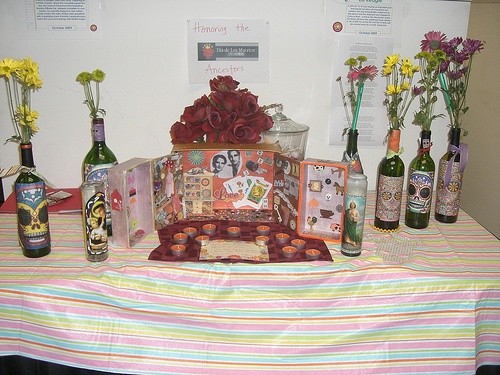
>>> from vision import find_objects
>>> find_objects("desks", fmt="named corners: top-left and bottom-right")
top-left (0, 188), bottom-right (499, 374)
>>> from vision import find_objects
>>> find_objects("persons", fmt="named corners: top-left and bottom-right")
top-left (212, 150), bottom-right (240, 178)
top-left (164, 163), bottom-right (175, 201)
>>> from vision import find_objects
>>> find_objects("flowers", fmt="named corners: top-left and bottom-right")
top-left (380, 31), bottom-right (486, 137)
top-left (336, 56), bottom-right (378, 138)
top-left (76, 70), bottom-right (106, 120)
top-left (0, 58), bottom-right (43, 145)
top-left (169, 74), bottom-right (274, 145)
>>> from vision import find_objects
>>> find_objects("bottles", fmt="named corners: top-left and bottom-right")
top-left (341, 174), bottom-right (368, 257)
top-left (80, 182), bottom-right (108, 261)
